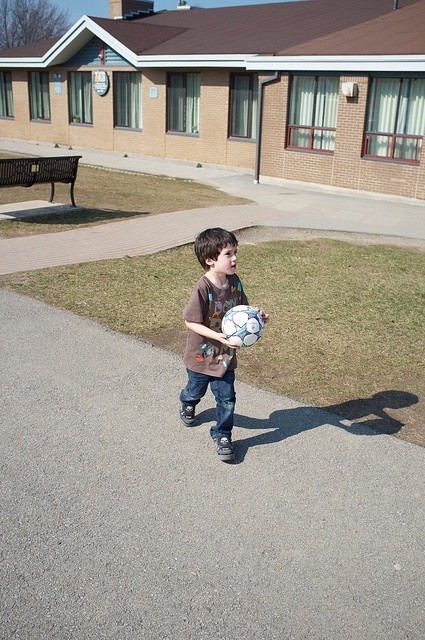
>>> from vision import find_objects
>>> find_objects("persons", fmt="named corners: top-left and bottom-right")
top-left (179, 227), bottom-right (271, 463)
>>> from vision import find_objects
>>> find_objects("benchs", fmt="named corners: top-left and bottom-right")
top-left (0, 155), bottom-right (83, 208)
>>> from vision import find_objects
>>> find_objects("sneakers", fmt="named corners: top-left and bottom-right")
top-left (214, 433), bottom-right (235, 460)
top-left (179, 402), bottom-right (195, 427)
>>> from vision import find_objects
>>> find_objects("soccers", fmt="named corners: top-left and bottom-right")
top-left (221, 305), bottom-right (264, 347)
